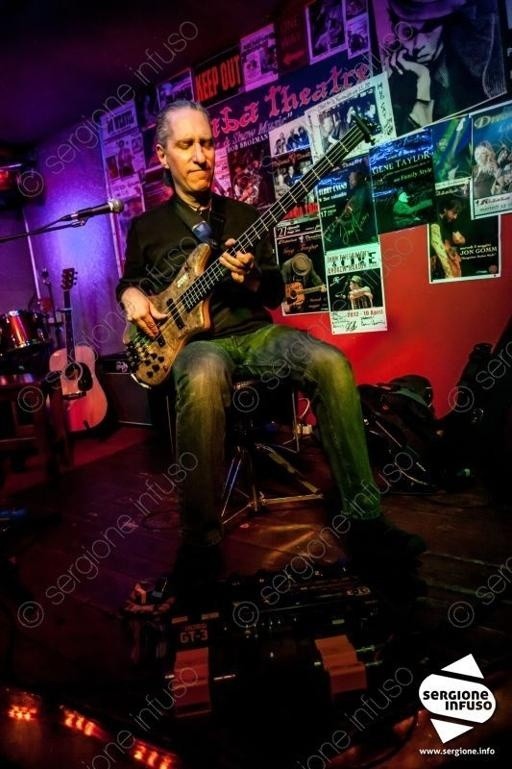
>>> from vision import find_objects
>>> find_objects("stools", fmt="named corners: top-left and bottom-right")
top-left (142, 371), bottom-right (327, 530)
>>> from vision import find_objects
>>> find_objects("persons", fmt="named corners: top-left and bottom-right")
top-left (379, 0), bottom-right (508, 137)
top-left (115, 139), bottom-right (136, 183)
top-left (117, 202), bottom-right (136, 240)
top-left (285, 129), bottom-right (300, 152)
top-left (473, 140), bottom-right (512, 201)
top-left (284, 164), bottom-right (302, 186)
top-left (326, 103), bottom-right (371, 146)
top-left (275, 132), bottom-right (286, 155)
top-left (230, 159), bottom-right (263, 206)
top-left (430, 199), bottom-right (498, 283)
top-left (279, 251), bottom-right (328, 315)
top-left (345, 272), bottom-right (374, 311)
top-left (114, 94), bottom-right (430, 612)
top-left (274, 172), bottom-right (291, 200)
top-left (292, 126), bottom-right (309, 147)
top-left (333, 168), bottom-right (377, 248)
top-left (390, 187), bottom-right (433, 230)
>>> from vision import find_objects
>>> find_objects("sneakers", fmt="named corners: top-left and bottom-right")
top-left (343, 516), bottom-right (426, 562)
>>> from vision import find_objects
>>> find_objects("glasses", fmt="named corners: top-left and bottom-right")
top-left (403, 19), bottom-right (443, 40)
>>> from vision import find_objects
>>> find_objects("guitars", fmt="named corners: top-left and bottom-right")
top-left (324, 198), bottom-right (353, 243)
top-left (48, 268), bottom-right (108, 434)
top-left (282, 281), bottom-right (327, 310)
top-left (442, 237), bottom-right (462, 277)
top-left (122, 113), bottom-right (380, 390)
top-left (0, 373), bottom-right (53, 471)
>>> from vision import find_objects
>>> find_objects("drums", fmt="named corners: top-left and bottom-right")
top-left (29, 311), bottom-right (52, 353)
top-left (1, 308), bottom-right (42, 361)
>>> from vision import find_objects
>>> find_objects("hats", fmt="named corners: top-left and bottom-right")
top-left (292, 253), bottom-right (312, 275)
top-left (389, 0), bottom-right (466, 19)
top-left (349, 275), bottom-right (363, 283)
top-left (389, 375), bottom-right (432, 407)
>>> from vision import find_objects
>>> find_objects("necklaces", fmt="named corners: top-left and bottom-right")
top-left (179, 194), bottom-right (213, 217)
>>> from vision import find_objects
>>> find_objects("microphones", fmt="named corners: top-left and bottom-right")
top-left (70, 198), bottom-right (124, 220)
